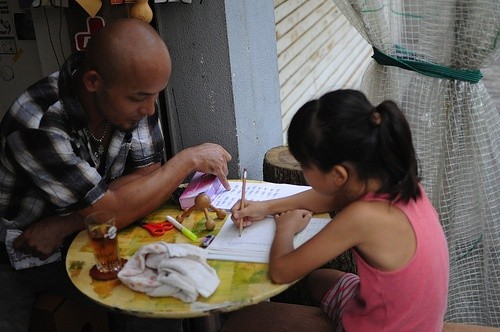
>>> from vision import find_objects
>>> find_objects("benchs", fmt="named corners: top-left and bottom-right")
top-left (220, 301), bottom-right (500, 332)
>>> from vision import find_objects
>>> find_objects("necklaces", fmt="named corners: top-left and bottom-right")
top-left (84, 119), bottom-right (109, 154)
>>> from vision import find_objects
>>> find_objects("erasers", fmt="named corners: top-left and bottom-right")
top-left (202, 234), bottom-right (215, 247)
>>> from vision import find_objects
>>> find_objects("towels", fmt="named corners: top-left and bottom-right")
top-left (117, 240), bottom-right (220, 302)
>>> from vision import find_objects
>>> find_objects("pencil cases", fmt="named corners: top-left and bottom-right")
top-left (179, 170), bottom-right (221, 209)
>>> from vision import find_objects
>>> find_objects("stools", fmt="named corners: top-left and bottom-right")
top-left (27, 296), bottom-right (111, 332)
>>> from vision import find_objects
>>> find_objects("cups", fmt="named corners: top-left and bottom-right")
top-left (85, 211), bottom-right (121, 273)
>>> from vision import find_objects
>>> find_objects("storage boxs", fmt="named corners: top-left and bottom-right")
top-left (179, 170), bottom-right (222, 210)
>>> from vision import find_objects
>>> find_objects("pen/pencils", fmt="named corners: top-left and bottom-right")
top-left (166, 215), bottom-right (197, 241)
top-left (239, 168), bottom-right (247, 237)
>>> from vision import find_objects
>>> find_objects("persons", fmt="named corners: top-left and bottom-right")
top-left (0, 18), bottom-right (232, 332)
top-left (231, 89), bottom-right (450, 332)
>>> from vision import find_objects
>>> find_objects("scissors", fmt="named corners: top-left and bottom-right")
top-left (136, 220), bottom-right (173, 237)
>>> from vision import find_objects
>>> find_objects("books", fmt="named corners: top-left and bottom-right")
top-left (209, 182), bottom-right (313, 212)
top-left (203, 214), bottom-right (329, 263)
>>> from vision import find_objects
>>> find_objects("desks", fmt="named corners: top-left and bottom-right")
top-left (66, 180), bottom-right (332, 332)
